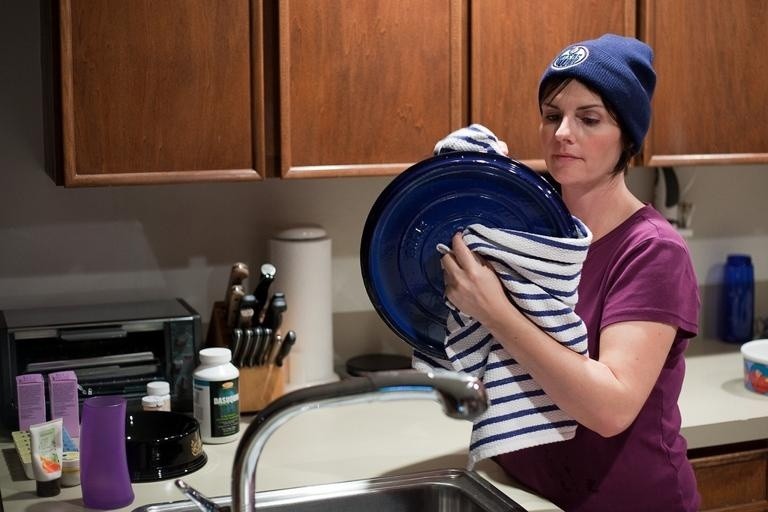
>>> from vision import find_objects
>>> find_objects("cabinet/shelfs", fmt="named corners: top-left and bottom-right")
top-left (469, 0), bottom-right (639, 170)
top-left (640, 0), bottom-right (768, 166)
top-left (273, 0), bottom-right (467, 180)
top-left (40, 1), bottom-right (276, 190)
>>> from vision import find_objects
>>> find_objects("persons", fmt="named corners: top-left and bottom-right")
top-left (439, 33), bottom-right (701, 512)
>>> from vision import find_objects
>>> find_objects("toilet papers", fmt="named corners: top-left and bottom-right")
top-left (269, 237), bottom-right (334, 379)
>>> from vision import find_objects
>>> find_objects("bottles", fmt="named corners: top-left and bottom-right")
top-left (142, 380), bottom-right (172, 411)
top-left (718, 253), bottom-right (756, 346)
top-left (192, 346), bottom-right (241, 444)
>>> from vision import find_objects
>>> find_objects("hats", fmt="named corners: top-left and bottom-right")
top-left (538, 31), bottom-right (657, 157)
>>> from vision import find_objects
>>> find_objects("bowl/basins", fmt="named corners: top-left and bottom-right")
top-left (125, 413), bottom-right (209, 483)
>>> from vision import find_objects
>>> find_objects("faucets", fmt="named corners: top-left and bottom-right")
top-left (231, 369), bottom-right (489, 511)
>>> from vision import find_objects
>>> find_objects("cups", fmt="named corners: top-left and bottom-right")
top-left (78, 392), bottom-right (137, 511)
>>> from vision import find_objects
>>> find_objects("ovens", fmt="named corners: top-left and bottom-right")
top-left (0, 297), bottom-right (204, 435)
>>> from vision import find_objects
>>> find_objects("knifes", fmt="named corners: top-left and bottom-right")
top-left (223, 262), bottom-right (297, 367)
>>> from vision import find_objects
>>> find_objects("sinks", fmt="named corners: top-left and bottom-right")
top-left (132, 468), bottom-right (527, 512)
